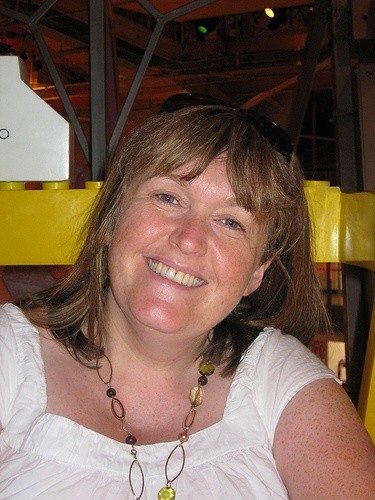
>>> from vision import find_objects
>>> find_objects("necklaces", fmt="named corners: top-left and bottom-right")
top-left (92, 315), bottom-right (216, 500)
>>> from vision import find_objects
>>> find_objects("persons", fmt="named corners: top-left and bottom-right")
top-left (1, 103), bottom-right (374, 500)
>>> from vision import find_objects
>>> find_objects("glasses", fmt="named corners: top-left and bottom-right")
top-left (158, 93), bottom-right (295, 164)
